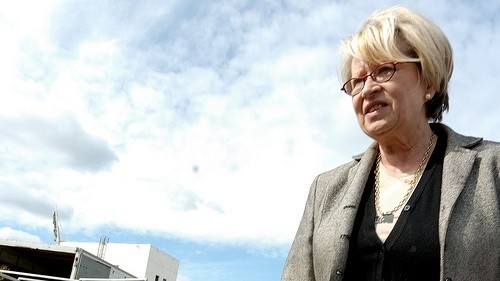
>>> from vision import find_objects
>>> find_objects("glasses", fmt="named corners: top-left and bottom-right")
top-left (341, 61), bottom-right (419, 96)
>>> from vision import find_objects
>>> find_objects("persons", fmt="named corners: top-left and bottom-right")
top-left (278, 6), bottom-right (500, 281)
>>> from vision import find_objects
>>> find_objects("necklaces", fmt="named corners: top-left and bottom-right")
top-left (374, 131), bottom-right (437, 223)
